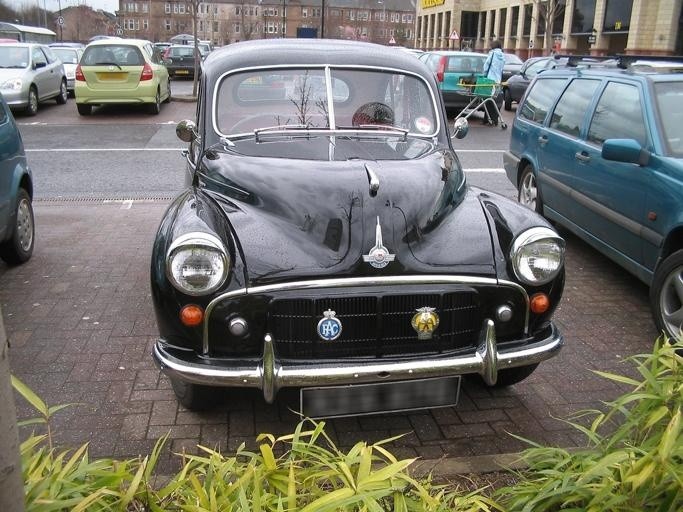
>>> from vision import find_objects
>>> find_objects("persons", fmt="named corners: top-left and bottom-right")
top-left (480, 39), bottom-right (505, 126)
top-left (551, 44), bottom-right (560, 56)
